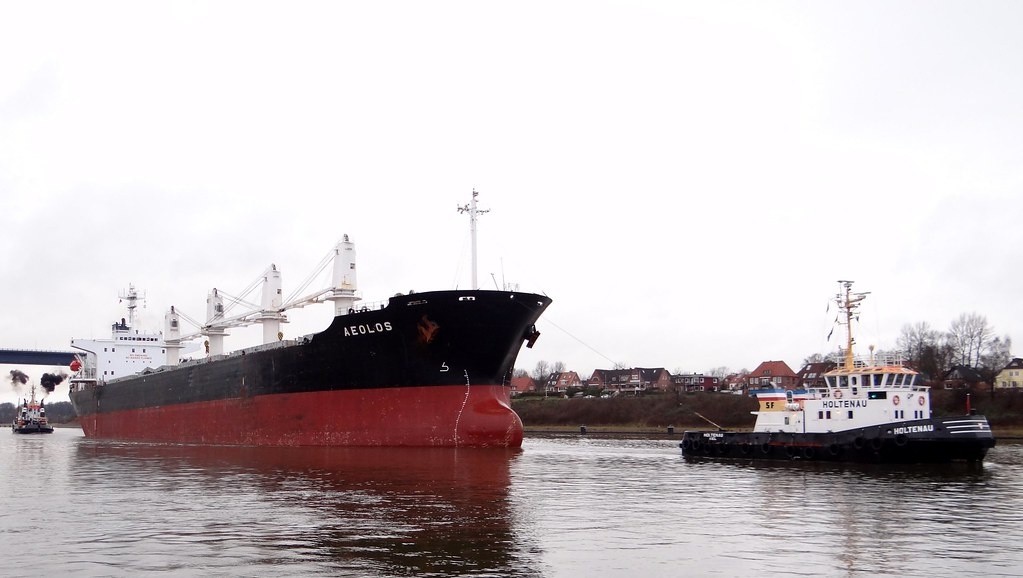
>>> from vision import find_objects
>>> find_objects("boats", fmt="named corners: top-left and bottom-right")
top-left (69, 188), bottom-right (553, 448)
top-left (12, 386), bottom-right (54, 434)
top-left (679, 279), bottom-right (996, 475)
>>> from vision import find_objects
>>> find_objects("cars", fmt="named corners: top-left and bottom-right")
top-left (601, 394), bottom-right (612, 398)
top-left (583, 394), bottom-right (596, 399)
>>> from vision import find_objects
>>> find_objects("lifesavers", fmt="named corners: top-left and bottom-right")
top-left (918, 396), bottom-right (925, 405)
top-left (893, 396), bottom-right (900, 404)
top-left (834, 390), bottom-right (842, 399)
